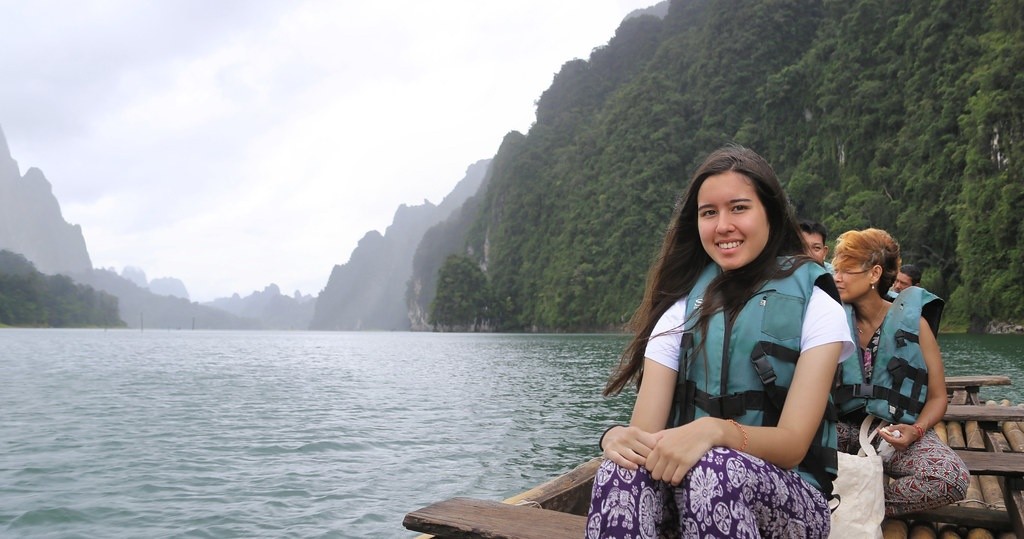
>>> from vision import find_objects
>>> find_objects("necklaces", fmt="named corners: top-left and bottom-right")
top-left (855, 304), bottom-right (886, 335)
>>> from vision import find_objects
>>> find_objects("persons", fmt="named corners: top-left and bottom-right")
top-left (584, 144), bottom-right (857, 539)
top-left (831, 228), bottom-right (970, 518)
top-left (889, 264), bottom-right (924, 295)
top-left (798, 222), bottom-right (834, 278)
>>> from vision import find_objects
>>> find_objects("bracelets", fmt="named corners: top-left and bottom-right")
top-left (727, 419), bottom-right (748, 452)
top-left (599, 424), bottom-right (624, 453)
top-left (911, 424), bottom-right (924, 441)
